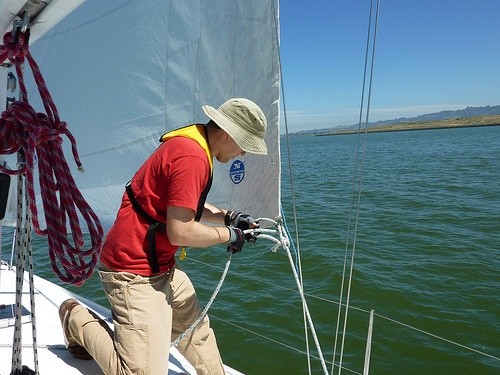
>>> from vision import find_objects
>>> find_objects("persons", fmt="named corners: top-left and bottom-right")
top-left (58, 97), bottom-right (267, 375)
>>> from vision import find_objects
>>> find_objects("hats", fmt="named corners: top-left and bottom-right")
top-left (203, 98), bottom-right (267, 155)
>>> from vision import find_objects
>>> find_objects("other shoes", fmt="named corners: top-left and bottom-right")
top-left (59, 299), bottom-right (93, 360)
